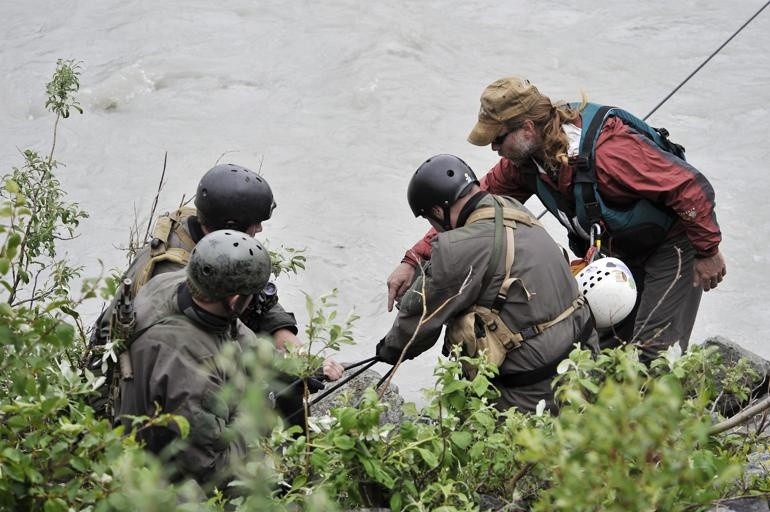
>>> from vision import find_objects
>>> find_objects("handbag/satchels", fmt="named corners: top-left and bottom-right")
top-left (444, 307), bottom-right (521, 380)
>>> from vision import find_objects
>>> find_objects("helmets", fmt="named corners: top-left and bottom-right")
top-left (575, 257), bottom-right (638, 328)
top-left (407, 153), bottom-right (481, 217)
top-left (186, 229), bottom-right (271, 299)
top-left (193, 164), bottom-right (276, 230)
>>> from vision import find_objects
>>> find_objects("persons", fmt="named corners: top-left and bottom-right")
top-left (376, 152), bottom-right (604, 424)
top-left (107, 226), bottom-right (345, 504)
top-left (387, 73), bottom-right (728, 377)
top-left (77, 160), bottom-right (346, 480)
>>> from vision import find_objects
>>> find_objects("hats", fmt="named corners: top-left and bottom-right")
top-left (468, 77), bottom-right (541, 146)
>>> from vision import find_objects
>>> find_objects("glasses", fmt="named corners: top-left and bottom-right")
top-left (494, 129), bottom-right (515, 145)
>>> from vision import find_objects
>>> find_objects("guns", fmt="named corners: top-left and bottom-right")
top-left (105, 278), bottom-right (136, 429)
